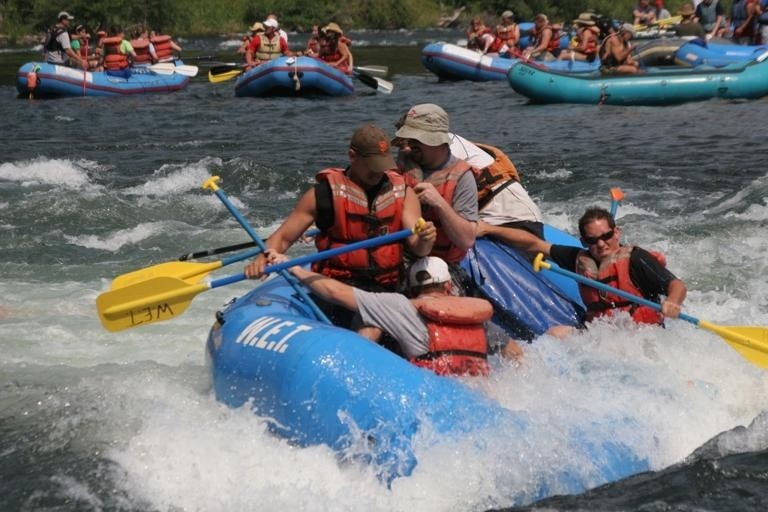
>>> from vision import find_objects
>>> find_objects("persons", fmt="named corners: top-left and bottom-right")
top-left (676, 0), bottom-right (768, 46)
top-left (476, 205), bottom-right (687, 339)
top-left (558, 12), bottom-right (598, 63)
top-left (237, 13), bottom-right (354, 74)
top-left (245, 124), bottom-right (439, 342)
top-left (523, 14), bottom-right (560, 63)
top-left (467, 10), bottom-right (521, 58)
top-left (598, 23), bottom-right (638, 73)
top-left (633, 0), bottom-right (672, 25)
top-left (395, 112), bottom-right (545, 261)
top-left (296, 103), bottom-right (479, 295)
top-left (40, 12), bottom-right (182, 71)
top-left (265, 248), bottom-right (522, 379)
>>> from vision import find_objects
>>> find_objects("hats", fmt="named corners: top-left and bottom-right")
top-left (676, 3), bottom-right (697, 15)
top-left (640, 0), bottom-right (663, 6)
top-left (621, 23), bottom-right (635, 41)
top-left (263, 18), bottom-right (278, 28)
top-left (248, 22), bottom-right (265, 32)
top-left (572, 12), bottom-right (596, 26)
top-left (410, 256), bottom-right (451, 287)
top-left (391, 103), bottom-right (454, 148)
top-left (349, 126), bottom-right (398, 173)
top-left (502, 10), bottom-right (518, 19)
top-left (534, 14), bottom-right (547, 23)
top-left (57, 11), bottom-right (74, 20)
top-left (321, 22), bottom-right (343, 38)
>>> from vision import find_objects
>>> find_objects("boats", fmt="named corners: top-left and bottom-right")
top-left (16, 59), bottom-right (189, 96)
top-left (620, 21), bottom-right (768, 72)
top-left (233, 56), bottom-right (355, 98)
top-left (420, 21), bottom-right (606, 80)
top-left (505, 44), bottom-right (768, 105)
top-left (204, 219), bottom-right (649, 505)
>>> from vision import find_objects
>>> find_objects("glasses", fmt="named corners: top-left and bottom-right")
top-left (582, 230), bottom-right (614, 245)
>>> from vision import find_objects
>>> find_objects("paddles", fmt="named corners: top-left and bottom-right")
top-left (353, 70), bottom-right (395, 94)
top-left (175, 65), bottom-right (198, 77)
top-left (532, 252), bottom-right (768, 368)
top-left (97, 217), bottom-right (427, 333)
top-left (208, 69), bottom-right (245, 84)
top-left (354, 66), bottom-right (388, 78)
top-left (110, 230), bottom-right (321, 285)
top-left (211, 62), bottom-right (261, 74)
top-left (306, 52), bottom-right (378, 89)
top-left (130, 63), bottom-right (174, 75)
